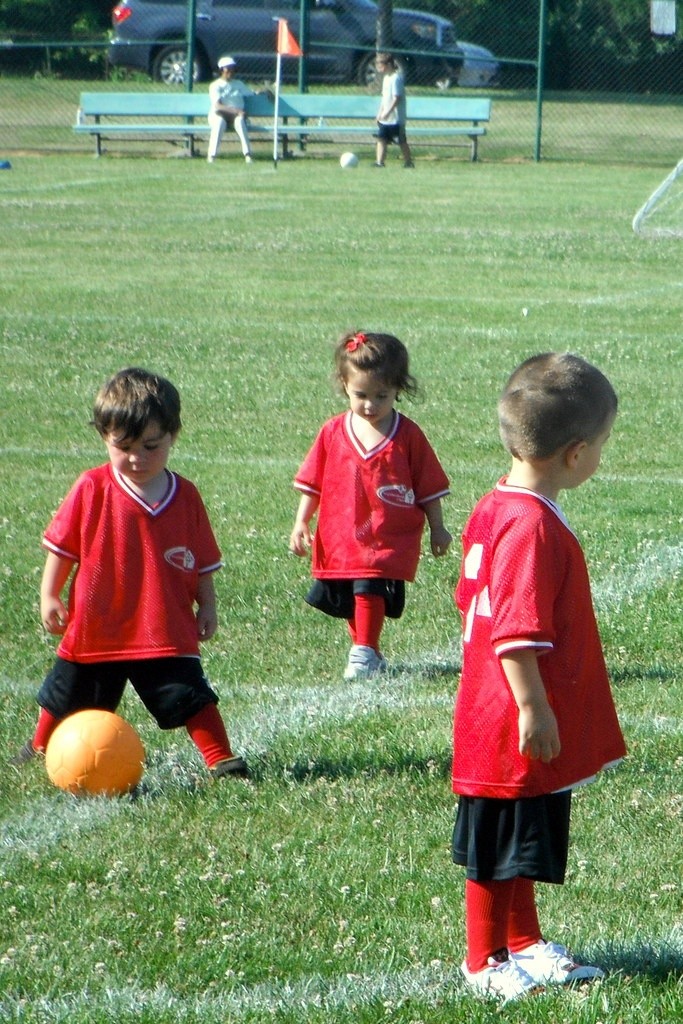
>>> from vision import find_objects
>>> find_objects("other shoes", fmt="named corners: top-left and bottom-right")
top-left (370, 163), bottom-right (385, 167)
top-left (208, 755), bottom-right (247, 781)
top-left (402, 162), bottom-right (413, 168)
top-left (245, 156), bottom-right (252, 164)
top-left (207, 156), bottom-right (214, 163)
top-left (6, 739), bottom-right (35, 767)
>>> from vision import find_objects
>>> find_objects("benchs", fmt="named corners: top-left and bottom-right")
top-left (72, 92), bottom-right (491, 163)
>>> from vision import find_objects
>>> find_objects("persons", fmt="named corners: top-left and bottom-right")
top-left (371, 52), bottom-right (414, 167)
top-left (207, 57), bottom-right (275, 162)
top-left (290, 330), bottom-right (453, 682)
top-left (9, 368), bottom-right (251, 780)
top-left (451, 353), bottom-right (626, 995)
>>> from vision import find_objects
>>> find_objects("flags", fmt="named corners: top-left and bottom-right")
top-left (281, 26), bottom-right (303, 56)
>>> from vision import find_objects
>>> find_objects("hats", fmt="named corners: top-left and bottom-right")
top-left (217, 57), bottom-right (237, 68)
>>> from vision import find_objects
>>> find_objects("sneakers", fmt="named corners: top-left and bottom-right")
top-left (343, 645), bottom-right (386, 682)
top-left (460, 949), bottom-right (558, 1014)
top-left (512, 939), bottom-right (607, 987)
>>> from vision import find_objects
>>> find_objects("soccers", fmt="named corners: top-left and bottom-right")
top-left (340, 152), bottom-right (359, 169)
top-left (44, 709), bottom-right (145, 798)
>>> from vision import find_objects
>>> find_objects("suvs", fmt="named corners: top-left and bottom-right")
top-left (109, 0), bottom-right (500, 90)
